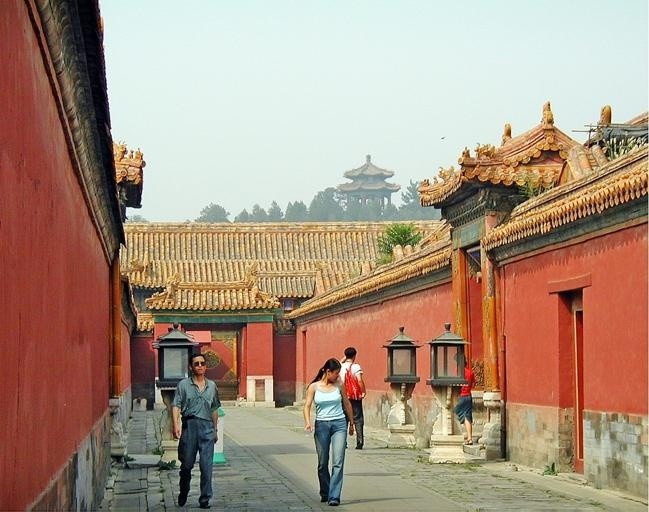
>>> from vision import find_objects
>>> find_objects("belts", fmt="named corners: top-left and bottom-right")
top-left (188, 416), bottom-right (204, 420)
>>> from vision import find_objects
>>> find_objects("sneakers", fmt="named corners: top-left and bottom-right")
top-left (321, 496), bottom-right (340, 506)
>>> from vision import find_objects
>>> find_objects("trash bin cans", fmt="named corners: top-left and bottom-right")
top-left (213, 408), bottom-right (225, 463)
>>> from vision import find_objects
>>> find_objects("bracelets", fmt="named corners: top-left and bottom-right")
top-left (350, 424), bottom-right (353, 427)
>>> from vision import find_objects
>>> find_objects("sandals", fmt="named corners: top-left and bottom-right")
top-left (463, 439), bottom-right (473, 445)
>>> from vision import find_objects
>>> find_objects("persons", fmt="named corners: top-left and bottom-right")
top-left (304, 358), bottom-right (355, 505)
top-left (338, 347), bottom-right (367, 449)
top-left (453, 353), bottom-right (476, 444)
top-left (172, 354), bottom-right (221, 508)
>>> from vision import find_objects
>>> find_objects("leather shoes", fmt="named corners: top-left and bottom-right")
top-left (200, 499), bottom-right (210, 508)
top-left (356, 441), bottom-right (363, 449)
top-left (179, 491), bottom-right (189, 506)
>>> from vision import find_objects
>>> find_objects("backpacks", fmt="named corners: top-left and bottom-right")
top-left (344, 364), bottom-right (362, 400)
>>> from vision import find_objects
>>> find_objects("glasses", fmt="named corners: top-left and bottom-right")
top-left (193, 361), bottom-right (206, 367)
